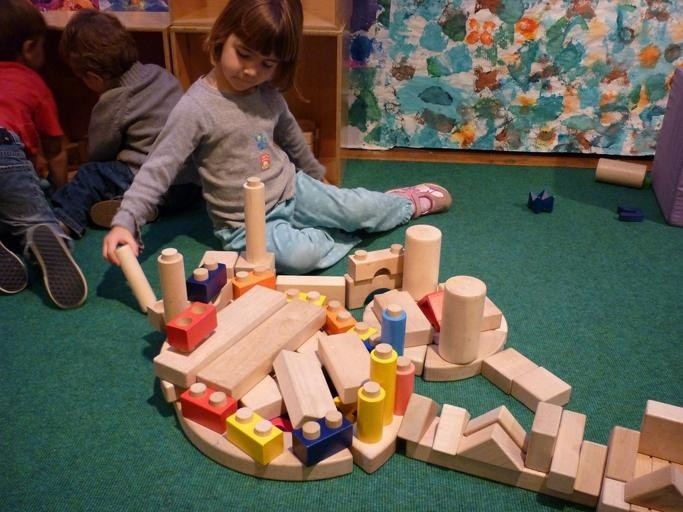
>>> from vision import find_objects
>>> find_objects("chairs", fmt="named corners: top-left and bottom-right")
top-left (384, 182), bottom-right (451, 218)
top-left (26, 223), bottom-right (87, 309)
top-left (0, 241), bottom-right (29, 294)
top-left (92, 200), bottom-right (158, 227)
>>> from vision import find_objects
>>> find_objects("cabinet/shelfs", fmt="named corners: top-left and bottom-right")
top-left (168, 0), bottom-right (354, 187)
top-left (40, 8), bottom-right (172, 175)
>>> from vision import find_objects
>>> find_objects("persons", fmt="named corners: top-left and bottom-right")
top-left (0, 0), bottom-right (89, 311)
top-left (49, 8), bottom-right (187, 240)
top-left (100, 0), bottom-right (455, 276)
